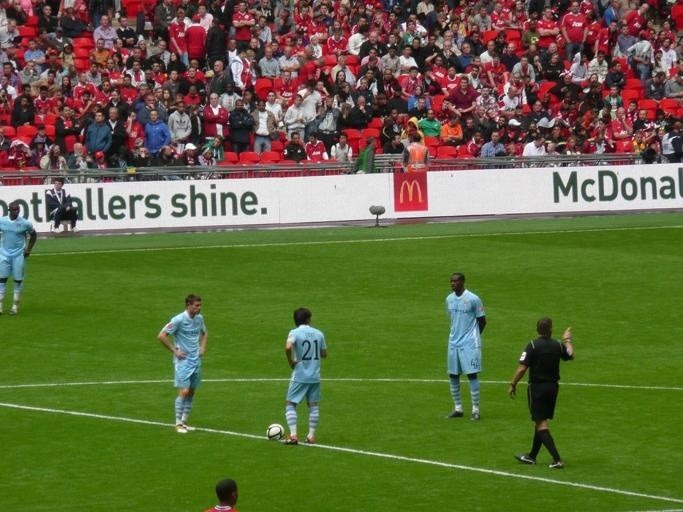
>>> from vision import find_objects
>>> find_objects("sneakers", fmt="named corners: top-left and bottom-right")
top-left (281, 436), bottom-right (298, 445)
top-left (9, 308), bottom-right (16, 315)
top-left (548, 461), bottom-right (564, 469)
top-left (305, 434), bottom-right (314, 444)
top-left (514, 454), bottom-right (536, 465)
top-left (183, 424), bottom-right (195, 432)
top-left (175, 425), bottom-right (188, 434)
top-left (471, 413), bottom-right (480, 421)
top-left (447, 411), bottom-right (463, 418)
top-left (72, 226), bottom-right (80, 233)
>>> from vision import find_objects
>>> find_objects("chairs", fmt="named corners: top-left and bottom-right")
top-left (0, 12), bottom-right (683, 184)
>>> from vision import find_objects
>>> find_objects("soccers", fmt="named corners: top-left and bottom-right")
top-left (267, 422), bottom-right (285, 439)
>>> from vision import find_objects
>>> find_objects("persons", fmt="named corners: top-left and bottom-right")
top-left (203, 479), bottom-right (240, 512)
top-left (443, 273), bottom-right (486, 421)
top-left (0, 200), bottom-right (36, 316)
top-left (506, 317), bottom-right (573, 469)
top-left (278, 307), bottom-right (327, 446)
top-left (45, 177), bottom-right (80, 234)
top-left (1, 0), bottom-right (683, 186)
top-left (157, 293), bottom-right (208, 435)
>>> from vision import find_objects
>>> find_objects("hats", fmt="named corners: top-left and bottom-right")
top-left (508, 119), bottom-right (521, 126)
top-left (201, 146), bottom-right (210, 155)
top-left (34, 137), bottom-right (45, 144)
top-left (94, 151), bottom-right (104, 161)
top-left (184, 142), bottom-right (197, 151)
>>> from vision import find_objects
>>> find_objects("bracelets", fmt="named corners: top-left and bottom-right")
top-left (562, 337), bottom-right (571, 343)
top-left (510, 383), bottom-right (514, 388)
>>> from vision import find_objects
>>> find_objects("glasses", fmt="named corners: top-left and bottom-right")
top-left (11, 208), bottom-right (19, 211)
